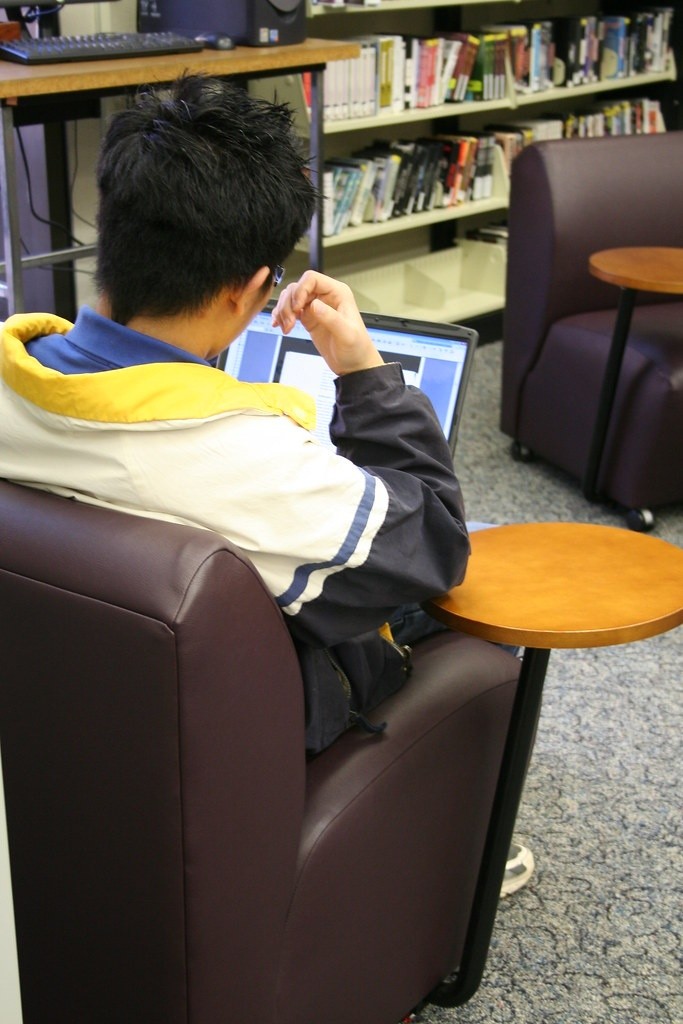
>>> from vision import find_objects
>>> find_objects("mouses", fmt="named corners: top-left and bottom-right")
top-left (194, 31), bottom-right (235, 49)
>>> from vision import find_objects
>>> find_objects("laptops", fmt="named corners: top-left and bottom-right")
top-left (215, 300), bottom-right (480, 464)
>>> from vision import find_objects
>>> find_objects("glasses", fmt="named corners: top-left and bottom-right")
top-left (240, 263), bottom-right (285, 288)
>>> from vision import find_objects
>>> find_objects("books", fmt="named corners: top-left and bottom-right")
top-left (302, 5), bottom-right (673, 123)
top-left (301, 98), bottom-right (668, 244)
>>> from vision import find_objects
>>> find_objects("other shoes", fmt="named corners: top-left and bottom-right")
top-left (499, 843), bottom-right (536, 898)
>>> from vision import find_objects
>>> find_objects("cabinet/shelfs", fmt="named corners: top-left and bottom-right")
top-left (53, 0), bottom-right (675, 340)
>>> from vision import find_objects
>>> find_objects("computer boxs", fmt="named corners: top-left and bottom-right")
top-left (135, 0), bottom-right (307, 48)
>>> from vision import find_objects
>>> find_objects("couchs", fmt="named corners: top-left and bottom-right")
top-left (0, 476), bottom-right (549, 1024)
top-left (486, 129), bottom-right (683, 507)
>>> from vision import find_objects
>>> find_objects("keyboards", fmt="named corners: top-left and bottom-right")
top-left (0, 31), bottom-right (204, 66)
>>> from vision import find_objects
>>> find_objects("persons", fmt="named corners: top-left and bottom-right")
top-left (0, 68), bottom-right (535, 900)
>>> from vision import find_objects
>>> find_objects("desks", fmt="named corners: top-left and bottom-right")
top-left (0, 28), bottom-right (364, 317)
top-left (581, 244), bottom-right (683, 504)
top-left (419, 517), bottom-right (683, 1008)
